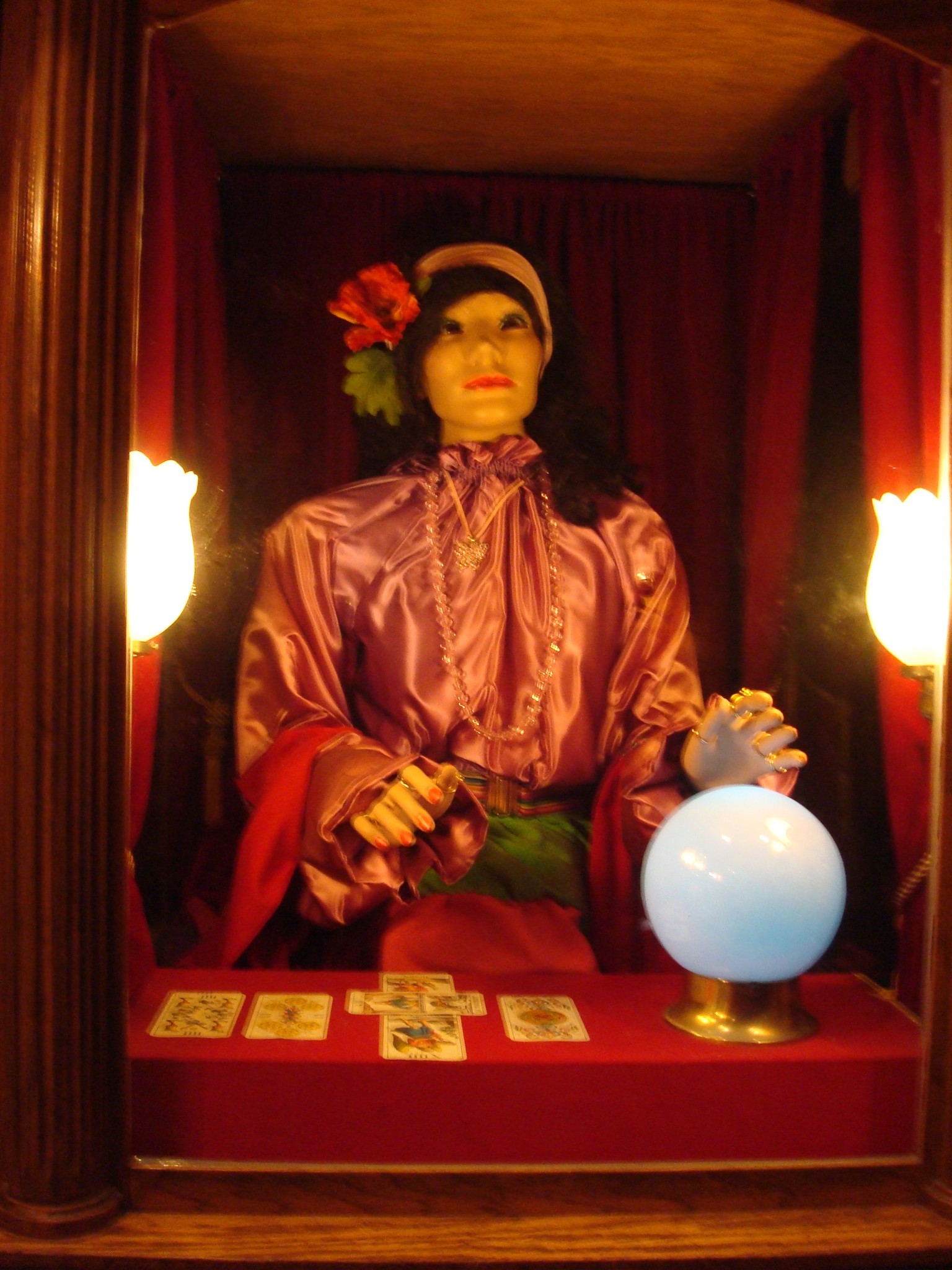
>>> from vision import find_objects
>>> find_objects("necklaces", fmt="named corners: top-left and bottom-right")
top-left (440, 466), bottom-right (526, 571)
top-left (414, 473), bottom-right (561, 746)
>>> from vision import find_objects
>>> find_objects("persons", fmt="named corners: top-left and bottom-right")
top-left (181, 225), bottom-right (810, 977)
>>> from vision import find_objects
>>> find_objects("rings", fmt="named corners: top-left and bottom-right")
top-left (396, 774), bottom-right (416, 791)
top-left (436, 770), bottom-right (465, 794)
top-left (691, 728), bottom-right (708, 745)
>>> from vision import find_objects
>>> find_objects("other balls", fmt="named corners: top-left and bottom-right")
top-left (641, 782), bottom-right (848, 983)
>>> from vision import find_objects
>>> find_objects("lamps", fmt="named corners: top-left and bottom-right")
top-left (865, 488), bottom-right (952, 722)
top-left (126, 450), bottom-right (197, 660)
